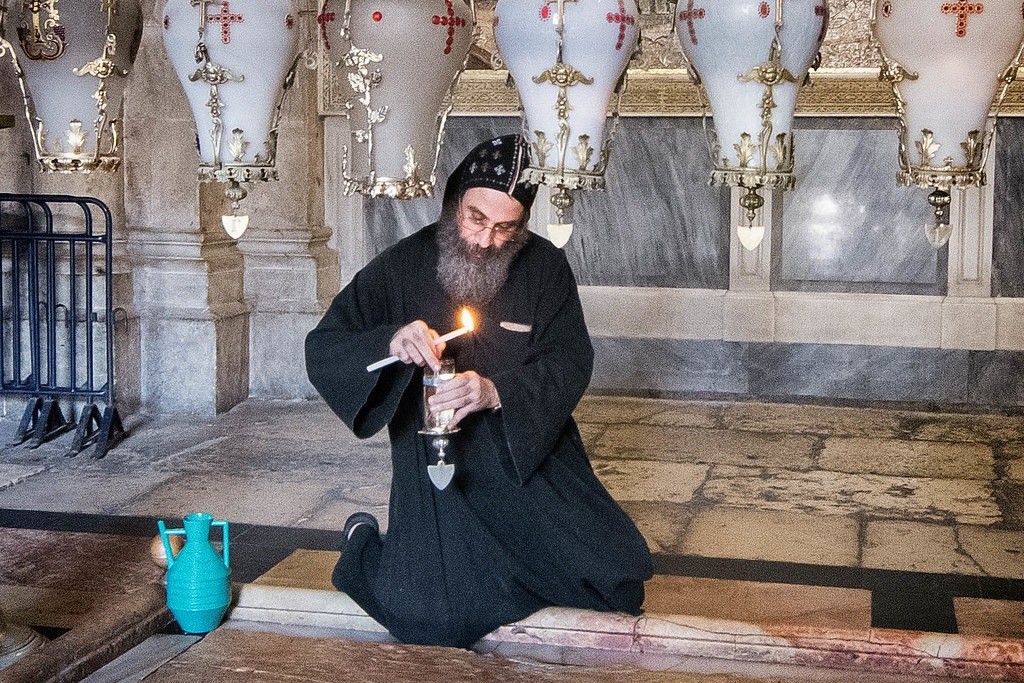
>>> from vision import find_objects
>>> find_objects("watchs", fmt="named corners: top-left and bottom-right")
top-left (492, 389), bottom-right (501, 414)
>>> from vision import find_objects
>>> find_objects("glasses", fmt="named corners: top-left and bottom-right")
top-left (457, 205), bottom-right (530, 244)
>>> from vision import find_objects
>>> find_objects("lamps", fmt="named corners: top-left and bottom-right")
top-left (659, 0), bottom-right (831, 251)
top-left (869, 0), bottom-right (1024, 249)
top-left (316, 0), bottom-right (483, 200)
top-left (161, 0), bottom-right (308, 239)
top-left (421, 360), bottom-right (460, 491)
top-left (0, 0), bottom-right (143, 176)
top-left (488, 0), bottom-right (643, 249)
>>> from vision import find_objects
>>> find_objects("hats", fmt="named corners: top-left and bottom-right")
top-left (459, 132), bottom-right (547, 210)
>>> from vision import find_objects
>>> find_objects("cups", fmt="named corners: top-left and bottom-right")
top-left (423, 361), bottom-right (456, 431)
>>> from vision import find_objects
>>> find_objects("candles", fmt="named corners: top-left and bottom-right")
top-left (366, 326), bottom-right (472, 372)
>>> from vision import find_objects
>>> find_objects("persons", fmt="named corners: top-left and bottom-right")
top-left (304, 135), bottom-right (654, 649)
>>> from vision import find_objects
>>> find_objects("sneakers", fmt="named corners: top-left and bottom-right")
top-left (343, 511), bottom-right (381, 553)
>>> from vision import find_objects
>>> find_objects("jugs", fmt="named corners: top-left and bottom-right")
top-left (157, 512), bottom-right (232, 634)
